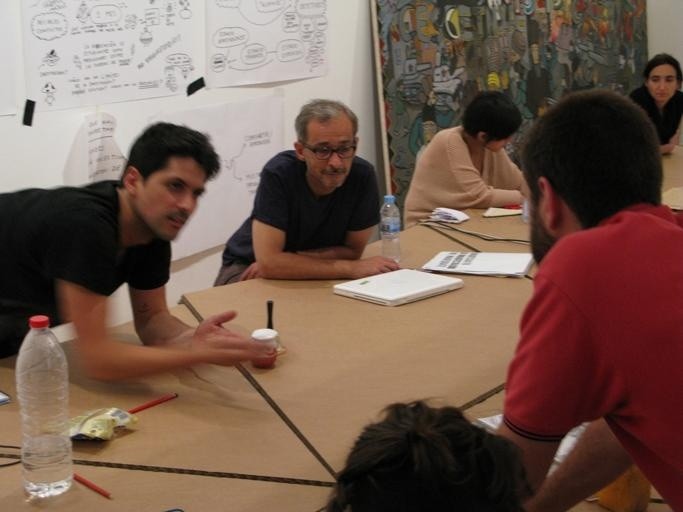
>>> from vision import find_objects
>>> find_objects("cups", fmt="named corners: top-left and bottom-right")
top-left (248, 328), bottom-right (277, 367)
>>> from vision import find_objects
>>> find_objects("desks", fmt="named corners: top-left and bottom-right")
top-left (0, 153), bottom-right (683, 512)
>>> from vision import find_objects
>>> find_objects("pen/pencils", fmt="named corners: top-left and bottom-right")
top-left (128, 393), bottom-right (179, 414)
top-left (73, 473), bottom-right (113, 499)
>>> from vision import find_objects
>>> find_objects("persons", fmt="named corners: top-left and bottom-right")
top-left (626, 50), bottom-right (683, 158)
top-left (312, 394), bottom-right (536, 509)
top-left (212, 95), bottom-right (400, 289)
top-left (0, 119), bottom-right (255, 385)
top-left (403, 89), bottom-right (526, 231)
top-left (487, 85), bottom-right (683, 511)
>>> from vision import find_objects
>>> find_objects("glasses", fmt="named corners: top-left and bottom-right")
top-left (300, 140), bottom-right (356, 161)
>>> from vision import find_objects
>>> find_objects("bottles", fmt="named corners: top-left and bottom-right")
top-left (14, 314), bottom-right (73, 499)
top-left (379, 193), bottom-right (402, 272)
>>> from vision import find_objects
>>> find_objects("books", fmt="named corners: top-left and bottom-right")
top-left (422, 248), bottom-right (535, 280)
top-left (331, 268), bottom-right (464, 306)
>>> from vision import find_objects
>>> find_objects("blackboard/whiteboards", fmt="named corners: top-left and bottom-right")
top-left (368, 0), bottom-right (648, 231)
